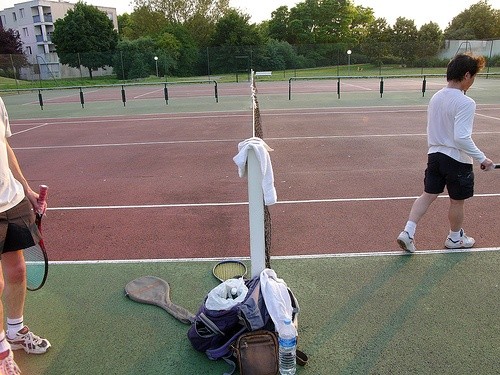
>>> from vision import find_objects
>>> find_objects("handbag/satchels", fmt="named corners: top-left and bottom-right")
top-left (230, 329), bottom-right (279, 375)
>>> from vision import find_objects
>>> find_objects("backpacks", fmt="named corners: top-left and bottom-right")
top-left (187, 275), bottom-right (299, 375)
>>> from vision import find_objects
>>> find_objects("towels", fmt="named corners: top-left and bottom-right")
top-left (232, 137), bottom-right (278, 206)
top-left (260, 268), bottom-right (299, 337)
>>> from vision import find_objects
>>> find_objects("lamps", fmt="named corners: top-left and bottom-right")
top-left (154, 56), bottom-right (159, 79)
top-left (347, 50), bottom-right (352, 65)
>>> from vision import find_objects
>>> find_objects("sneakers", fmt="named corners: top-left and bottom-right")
top-left (444, 228), bottom-right (476, 248)
top-left (396, 231), bottom-right (416, 253)
top-left (3, 324), bottom-right (52, 355)
top-left (0, 347), bottom-right (23, 375)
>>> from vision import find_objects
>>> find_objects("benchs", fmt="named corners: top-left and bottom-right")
top-left (254, 71), bottom-right (272, 78)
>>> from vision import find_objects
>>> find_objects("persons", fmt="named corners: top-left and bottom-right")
top-left (395, 53), bottom-right (494, 254)
top-left (0, 96), bottom-right (52, 375)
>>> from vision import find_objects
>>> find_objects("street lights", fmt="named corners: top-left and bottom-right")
top-left (346, 49), bottom-right (352, 66)
top-left (155, 56), bottom-right (159, 77)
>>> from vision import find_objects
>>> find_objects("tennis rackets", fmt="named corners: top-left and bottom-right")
top-left (212, 261), bottom-right (247, 283)
top-left (481, 164), bottom-right (500, 170)
top-left (23, 185), bottom-right (49, 291)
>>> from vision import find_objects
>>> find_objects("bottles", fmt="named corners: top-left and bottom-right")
top-left (278, 318), bottom-right (296, 375)
